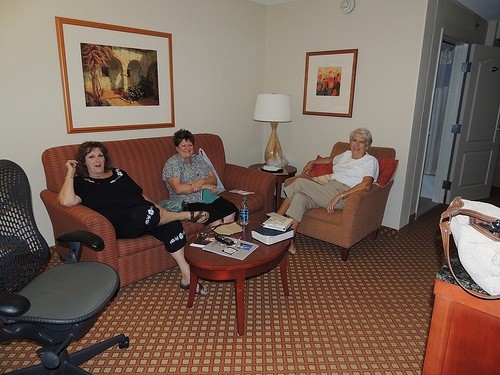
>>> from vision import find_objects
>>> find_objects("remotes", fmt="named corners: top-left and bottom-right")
top-left (214, 235), bottom-right (234, 245)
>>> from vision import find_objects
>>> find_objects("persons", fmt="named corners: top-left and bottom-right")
top-left (162, 128), bottom-right (239, 228)
top-left (276, 128), bottom-right (380, 254)
top-left (316, 70), bottom-right (342, 96)
top-left (57, 140), bottom-right (212, 296)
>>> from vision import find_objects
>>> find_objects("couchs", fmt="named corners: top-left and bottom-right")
top-left (278, 142), bottom-right (395, 261)
top-left (40, 134), bottom-right (278, 289)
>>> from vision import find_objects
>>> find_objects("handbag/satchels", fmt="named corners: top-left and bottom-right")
top-left (438, 194), bottom-right (500, 299)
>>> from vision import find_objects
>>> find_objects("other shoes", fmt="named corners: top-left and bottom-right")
top-left (288, 247), bottom-right (296, 254)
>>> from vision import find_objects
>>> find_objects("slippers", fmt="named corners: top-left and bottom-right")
top-left (180, 210), bottom-right (209, 224)
top-left (180, 279), bottom-right (209, 296)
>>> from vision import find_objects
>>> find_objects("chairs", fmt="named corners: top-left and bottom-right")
top-left (0, 160), bottom-right (132, 375)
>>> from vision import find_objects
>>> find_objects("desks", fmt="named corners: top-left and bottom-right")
top-left (419, 254), bottom-right (500, 375)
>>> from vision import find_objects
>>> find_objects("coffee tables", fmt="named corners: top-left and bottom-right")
top-left (184, 219), bottom-right (288, 335)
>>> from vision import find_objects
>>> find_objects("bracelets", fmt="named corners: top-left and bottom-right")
top-left (341, 192), bottom-right (344, 200)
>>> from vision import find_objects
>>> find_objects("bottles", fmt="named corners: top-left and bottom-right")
top-left (239, 197), bottom-right (250, 225)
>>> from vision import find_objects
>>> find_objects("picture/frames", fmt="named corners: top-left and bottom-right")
top-left (54, 16), bottom-right (175, 133)
top-left (302, 49), bottom-right (358, 118)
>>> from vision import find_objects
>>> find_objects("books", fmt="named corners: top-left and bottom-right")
top-left (190, 211), bottom-right (294, 260)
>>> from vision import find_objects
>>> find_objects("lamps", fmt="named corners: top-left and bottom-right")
top-left (254, 93), bottom-right (293, 165)
